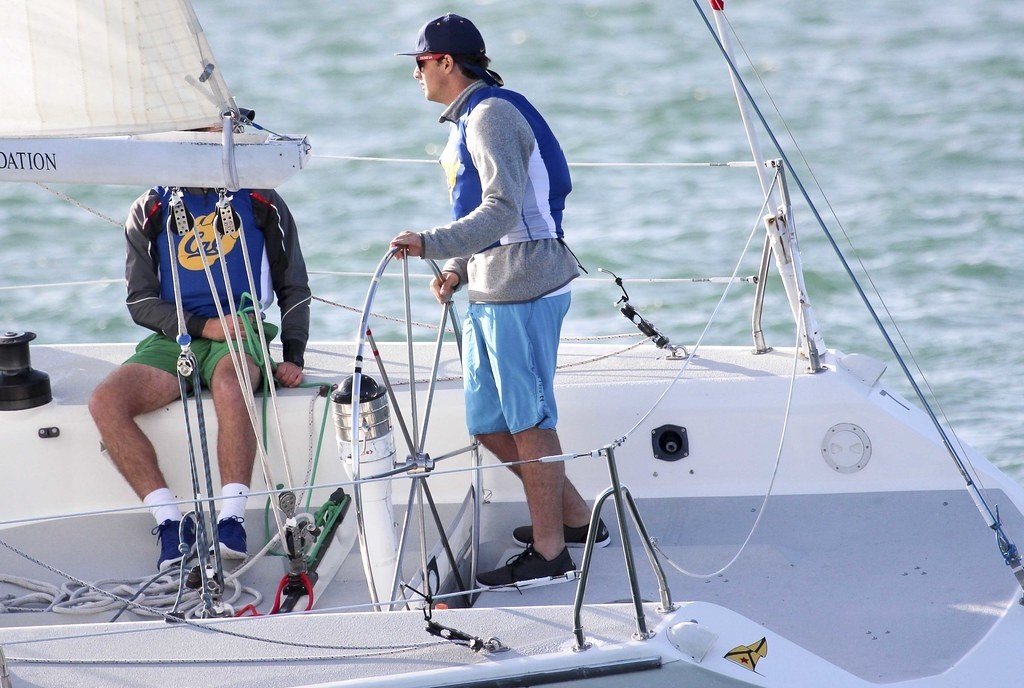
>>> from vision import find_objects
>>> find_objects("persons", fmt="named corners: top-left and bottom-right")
top-left (86, 130), bottom-right (311, 574)
top-left (389, 13), bottom-right (609, 592)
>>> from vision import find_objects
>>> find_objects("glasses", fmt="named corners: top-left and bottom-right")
top-left (416, 53), bottom-right (455, 72)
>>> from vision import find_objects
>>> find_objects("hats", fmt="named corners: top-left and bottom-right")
top-left (225, 106), bottom-right (256, 125)
top-left (394, 14), bottom-right (485, 55)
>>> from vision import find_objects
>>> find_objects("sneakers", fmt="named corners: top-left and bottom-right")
top-left (151, 515), bottom-right (199, 572)
top-left (474, 540), bottom-right (579, 593)
top-left (512, 517), bottom-right (610, 549)
top-left (215, 515), bottom-right (247, 560)
